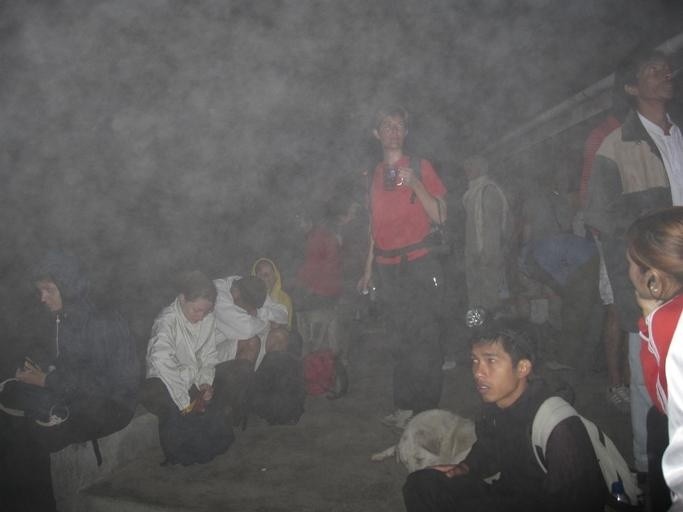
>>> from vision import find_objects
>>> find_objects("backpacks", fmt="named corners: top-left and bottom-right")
top-left (529, 393), bottom-right (643, 507)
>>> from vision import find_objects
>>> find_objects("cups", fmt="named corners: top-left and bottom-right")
top-left (380, 165), bottom-right (404, 193)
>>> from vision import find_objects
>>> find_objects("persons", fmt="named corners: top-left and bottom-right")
top-left (3, 47), bottom-right (681, 509)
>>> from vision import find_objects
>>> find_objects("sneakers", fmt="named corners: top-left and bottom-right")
top-left (378, 405), bottom-right (419, 435)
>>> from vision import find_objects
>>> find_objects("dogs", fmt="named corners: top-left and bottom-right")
top-left (370, 408), bottom-right (476, 476)
